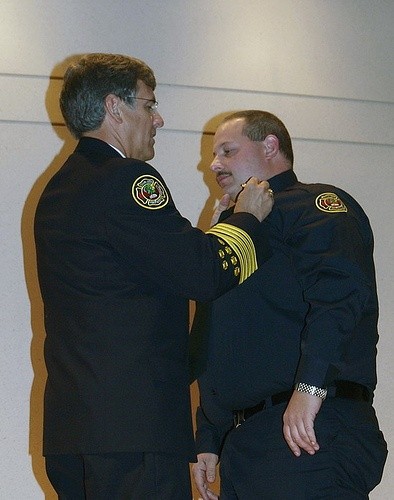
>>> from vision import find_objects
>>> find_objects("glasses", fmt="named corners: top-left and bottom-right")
top-left (101, 93), bottom-right (159, 112)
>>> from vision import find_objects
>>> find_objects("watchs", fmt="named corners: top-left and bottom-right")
top-left (295, 383), bottom-right (327, 401)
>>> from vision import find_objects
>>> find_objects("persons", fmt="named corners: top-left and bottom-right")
top-left (33, 52), bottom-right (274, 500)
top-left (190, 110), bottom-right (388, 500)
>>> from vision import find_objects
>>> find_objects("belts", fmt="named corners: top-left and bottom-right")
top-left (232, 380), bottom-right (373, 427)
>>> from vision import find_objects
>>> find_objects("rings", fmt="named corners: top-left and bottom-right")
top-left (268, 189), bottom-right (273, 196)
